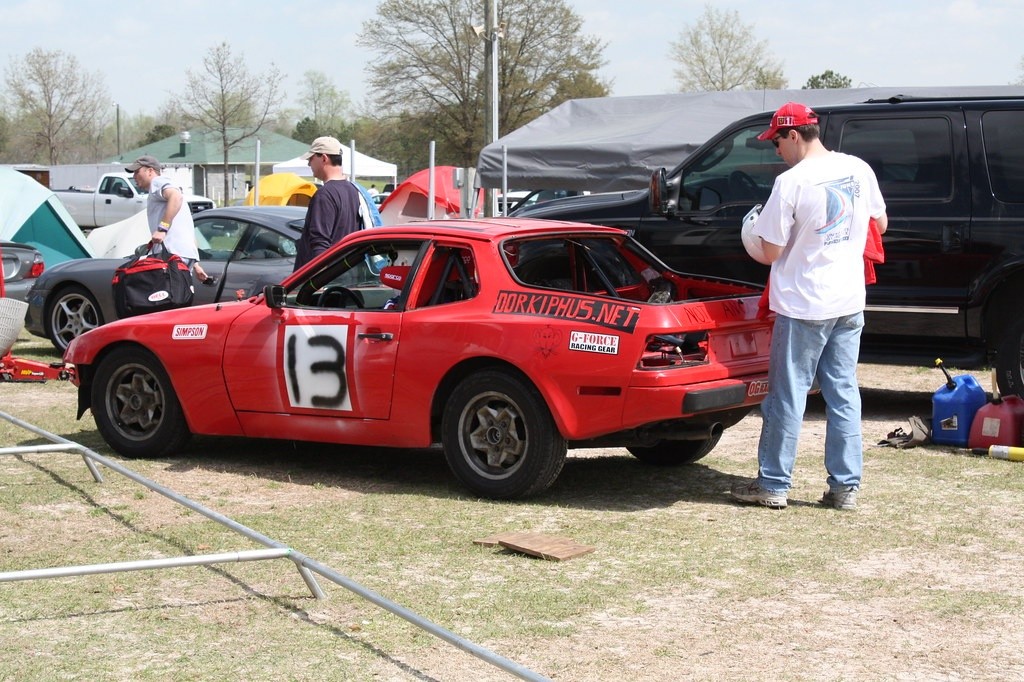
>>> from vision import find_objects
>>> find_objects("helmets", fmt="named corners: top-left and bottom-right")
top-left (741, 204), bottom-right (770, 265)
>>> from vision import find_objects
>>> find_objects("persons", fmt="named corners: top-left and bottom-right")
top-left (367, 184), bottom-right (379, 201)
top-left (731, 100), bottom-right (888, 508)
top-left (125, 155), bottom-right (207, 282)
top-left (295, 138), bottom-right (363, 291)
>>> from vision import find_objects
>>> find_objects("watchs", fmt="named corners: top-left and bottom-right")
top-left (158, 227), bottom-right (167, 232)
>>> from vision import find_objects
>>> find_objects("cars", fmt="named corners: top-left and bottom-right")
top-left (497, 188), bottom-right (590, 214)
top-left (62, 218), bottom-right (822, 499)
top-left (0, 240), bottom-right (44, 303)
top-left (372, 192), bottom-right (391, 209)
top-left (25, 205), bottom-right (371, 355)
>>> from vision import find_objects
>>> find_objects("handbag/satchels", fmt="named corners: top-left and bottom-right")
top-left (112, 240), bottom-right (195, 321)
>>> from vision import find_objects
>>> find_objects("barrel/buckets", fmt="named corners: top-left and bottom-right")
top-left (968, 367), bottom-right (1024, 451)
top-left (932, 357), bottom-right (987, 448)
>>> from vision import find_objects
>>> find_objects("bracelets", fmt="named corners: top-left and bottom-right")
top-left (159, 221), bottom-right (170, 230)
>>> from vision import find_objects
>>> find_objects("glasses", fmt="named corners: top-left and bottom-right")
top-left (772, 135), bottom-right (785, 146)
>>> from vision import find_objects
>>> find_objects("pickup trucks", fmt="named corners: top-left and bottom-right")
top-left (54, 171), bottom-right (218, 230)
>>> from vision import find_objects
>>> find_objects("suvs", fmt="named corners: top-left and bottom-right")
top-left (507, 85), bottom-right (1024, 397)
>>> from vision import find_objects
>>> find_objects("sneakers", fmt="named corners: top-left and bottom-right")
top-left (818, 482), bottom-right (860, 512)
top-left (731, 476), bottom-right (789, 507)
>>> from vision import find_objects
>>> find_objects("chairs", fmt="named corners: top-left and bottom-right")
top-left (252, 232), bottom-right (280, 253)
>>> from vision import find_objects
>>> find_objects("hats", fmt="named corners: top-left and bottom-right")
top-left (124, 155), bottom-right (161, 175)
top-left (304, 137), bottom-right (344, 159)
top-left (756, 102), bottom-right (818, 141)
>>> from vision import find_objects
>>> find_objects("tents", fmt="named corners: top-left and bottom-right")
top-left (470, 86), bottom-right (1024, 217)
top-left (273, 145), bottom-right (397, 189)
top-left (377, 166), bottom-right (485, 226)
top-left (0, 168), bottom-right (95, 270)
top-left (244, 173), bottom-right (317, 207)
